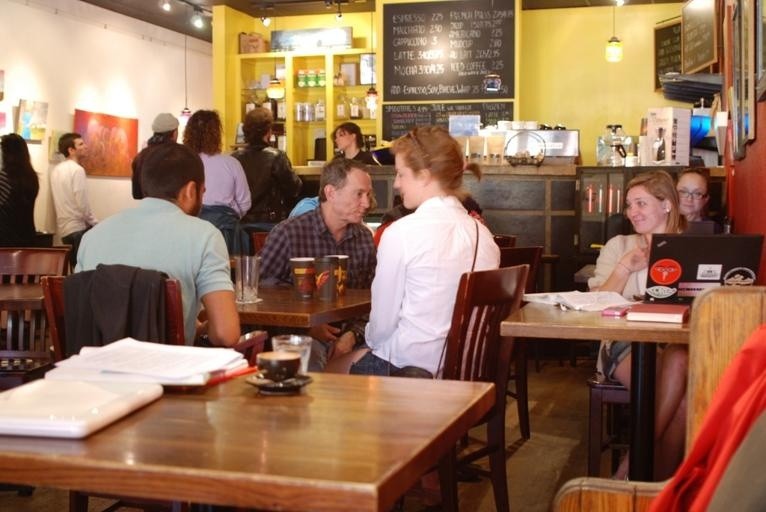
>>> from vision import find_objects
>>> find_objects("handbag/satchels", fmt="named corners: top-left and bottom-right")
top-left (387, 220), bottom-right (480, 378)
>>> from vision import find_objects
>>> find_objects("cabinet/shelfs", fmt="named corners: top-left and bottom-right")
top-left (234, 47), bottom-right (382, 169)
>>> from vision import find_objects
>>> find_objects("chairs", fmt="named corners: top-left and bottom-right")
top-left (1, 245), bottom-right (71, 386)
top-left (546, 379), bottom-right (766, 512)
top-left (497, 244), bottom-right (546, 440)
top-left (39, 272), bottom-right (266, 368)
top-left (683, 288), bottom-right (766, 482)
top-left (384, 262), bottom-right (532, 511)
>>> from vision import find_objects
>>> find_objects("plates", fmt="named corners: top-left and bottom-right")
top-left (245, 371), bottom-right (313, 395)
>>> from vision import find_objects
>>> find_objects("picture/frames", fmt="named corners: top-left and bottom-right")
top-left (740, 2), bottom-right (757, 147)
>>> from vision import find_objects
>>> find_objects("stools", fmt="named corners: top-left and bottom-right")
top-left (589, 358), bottom-right (631, 484)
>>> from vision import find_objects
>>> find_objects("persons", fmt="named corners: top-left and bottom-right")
top-left (132, 112), bottom-right (178, 200)
top-left (231, 108), bottom-right (303, 256)
top-left (0, 134), bottom-right (38, 368)
top-left (588, 170), bottom-right (688, 480)
top-left (255, 159), bottom-right (377, 374)
top-left (374, 192), bottom-right (484, 251)
top-left (286, 193), bottom-right (377, 241)
top-left (75, 141), bottom-right (241, 347)
top-left (674, 167), bottom-right (725, 238)
top-left (181, 110), bottom-right (250, 255)
top-left (331, 124), bottom-right (408, 164)
top-left (51, 133), bottom-right (96, 274)
top-left (323, 127), bottom-right (502, 375)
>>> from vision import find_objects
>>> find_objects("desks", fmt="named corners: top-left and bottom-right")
top-left (1, 371), bottom-right (495, 512)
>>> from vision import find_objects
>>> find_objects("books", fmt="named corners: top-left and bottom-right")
top-left (625, 304), bottom-right (690, 323)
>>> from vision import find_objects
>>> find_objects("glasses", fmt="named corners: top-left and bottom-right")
top-left (677, 188), bottom-right (708, 201)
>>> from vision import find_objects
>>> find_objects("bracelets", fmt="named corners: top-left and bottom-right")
top-left (621, 264), bottom-right (632, 273)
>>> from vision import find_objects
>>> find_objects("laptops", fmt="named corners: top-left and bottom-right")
top-left (631, 232), bottom-right (765, 305)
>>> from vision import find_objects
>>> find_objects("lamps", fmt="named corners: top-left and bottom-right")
top-left (605, 1), bottom-right (623, 64)
top-left (175, 3), bottom-right (193, 127)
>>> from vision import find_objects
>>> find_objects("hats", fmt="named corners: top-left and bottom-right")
top-left (150, 113), bottom-right (179, 133)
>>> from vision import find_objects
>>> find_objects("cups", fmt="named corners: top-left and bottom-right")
top-left (313, 257), bottom-right (340, 304)
top-left (270, 333), bottom-right (312, 373)
top-left (290, 257), bottom-right (315, 301)
top-left (325, 255), bottom-right (350, 297)
top-left (233, 254), bottom-right (261, 304)
top-left (255, 351), bottom-right (302, 384)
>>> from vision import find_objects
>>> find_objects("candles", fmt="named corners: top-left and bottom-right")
top-left (588, 188), bottom-right (593, 212)
top-left (599, 189), bottom-right (602, 212)
top-left (609, 188), bottom-right (613, 214)
top-left (616, 189), bottom-right (620, 213)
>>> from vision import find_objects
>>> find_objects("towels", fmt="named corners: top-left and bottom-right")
top-left (0, 376), bottom-right (164, 437)
